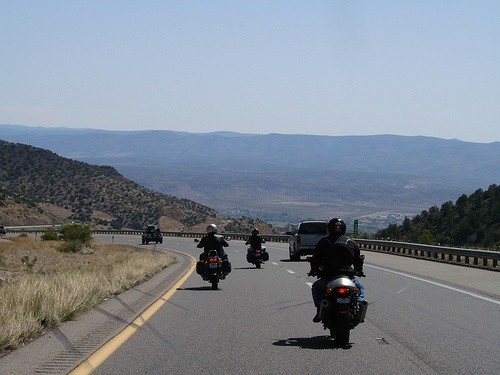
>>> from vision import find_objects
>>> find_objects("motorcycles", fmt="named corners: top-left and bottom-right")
top-left (245, 239), bottom-right (266, 268)
top-left (193, 236), bottom-right (231, 289)
top-left (305, 254), bottom-right (367, 345)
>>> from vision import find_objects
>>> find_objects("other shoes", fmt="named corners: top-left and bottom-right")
top-left (313, 314), bottom-right (321, 322)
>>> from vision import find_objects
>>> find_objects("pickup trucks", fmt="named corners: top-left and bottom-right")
top-left (142, 225), bottom-right (163, 245)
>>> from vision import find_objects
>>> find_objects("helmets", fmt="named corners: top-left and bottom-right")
top-left (327, 217), bottom-right (346, 236)
top-left (252, 229), bottom-right (259, 236)
top-left (207, 224), bottom-right (217, 233)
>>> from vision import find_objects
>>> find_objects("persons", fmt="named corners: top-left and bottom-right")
top-left (197, 224), bottom-right (229, 261)
top-left (308, 217), bottom-right (366, 323)
top-left (245, 229), bottom-right (265, 251)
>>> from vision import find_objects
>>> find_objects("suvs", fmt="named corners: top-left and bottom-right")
top-left (0, 225), bottom-right (6, 235)
top-left (286, 219), bottom-right (329, 261)
